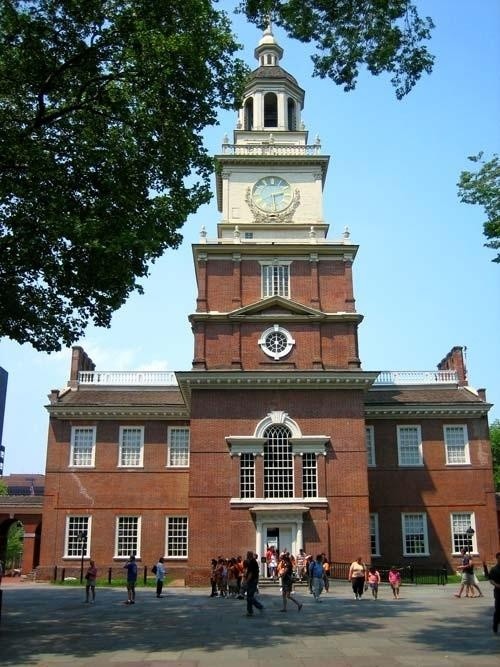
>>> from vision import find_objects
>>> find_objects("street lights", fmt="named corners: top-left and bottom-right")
top-left (466, 525), bottom-right (475, 553)
top-left (77, 532), bottom-right (87, 583)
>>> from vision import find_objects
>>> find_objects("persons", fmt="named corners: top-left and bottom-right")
top-left (464, 554), bottom-right (477, 597)
top-left (243, 549), bottom-right (265, 616)
top-left (208, 553), bottom-right (261, 599)
top-left (488, 550), bottom-right (500, 636)
top-left (123, 554), bottom-right (139, 604)
top-left (154, 556), bottom-right (169, 598)
top-left (279, 554), bottom-right (303, 613)
top-left (349, 555), bottom-right (367, 600)
top-left (367, 565), bottom-right (381, 600)
top-left (85, 559), bottom-right (98, 604)
top-left (453, 547), bottom-right (484, 598)
top-left (266, 544), bottom-right (331, 602)
top-left (388, 565), bottom-right (403, 599)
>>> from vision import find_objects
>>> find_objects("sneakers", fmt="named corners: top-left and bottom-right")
top-left (210, 593), bottom-right (244, 600)
top-left (129, 600), bottom-right (135, 604)
top-left (355, 596), bottom-right (402, 601)
top-left (123, 600), bottom-right (129, 605)
top-left (454, 593), bottom-right (484, 598)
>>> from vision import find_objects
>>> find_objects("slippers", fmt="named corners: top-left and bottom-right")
top-left (298, 604), bottom-right (302, 611)
top-left (279, 608), bottom-right (287, 612)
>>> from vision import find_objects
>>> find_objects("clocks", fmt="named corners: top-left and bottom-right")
top-left (251, 175), bottom-right (294, 213)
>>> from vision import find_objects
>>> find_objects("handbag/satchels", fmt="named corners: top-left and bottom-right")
top-left (151, 565), bottom-right (156, 574)
